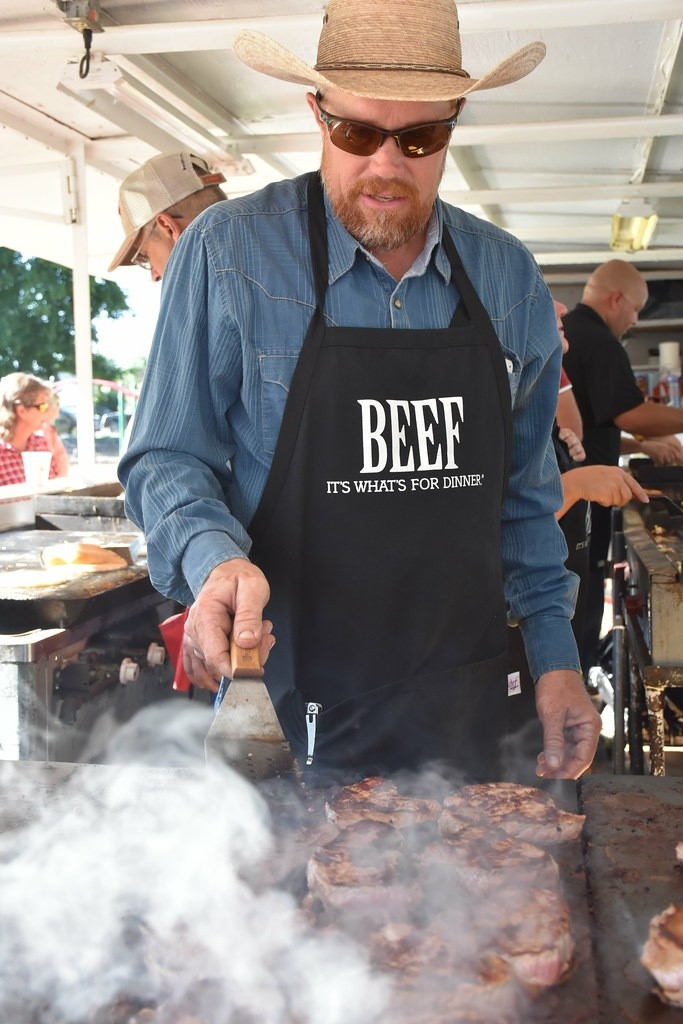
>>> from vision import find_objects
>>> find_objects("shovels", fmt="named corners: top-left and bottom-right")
top-left (637, 488), bottom-right (683, 518)
top-left (198, 629), bottom-right (304, 821)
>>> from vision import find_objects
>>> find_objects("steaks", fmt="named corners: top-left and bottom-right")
top-left (292, 777), bottom-right (683, 1020)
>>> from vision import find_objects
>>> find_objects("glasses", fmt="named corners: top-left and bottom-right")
top-left (131, 214), bottom-right (183, 270)
top-left (315, 90), bottom-right (462, 159)
top-left (24, 402), bottom-right (48, 413)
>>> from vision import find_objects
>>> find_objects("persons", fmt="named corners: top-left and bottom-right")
top-left (0, 152), bottom-right (683, 761)
top-left (118, 0), bottom-right (604, 781)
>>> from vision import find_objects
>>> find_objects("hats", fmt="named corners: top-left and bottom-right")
top-left (108, 152), bottom-right (226, 275)
top-left (234, 1), bottom-right (545, 103)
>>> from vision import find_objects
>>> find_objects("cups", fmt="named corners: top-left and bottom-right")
top-left (21, 451), bottom-right (53, 487)
